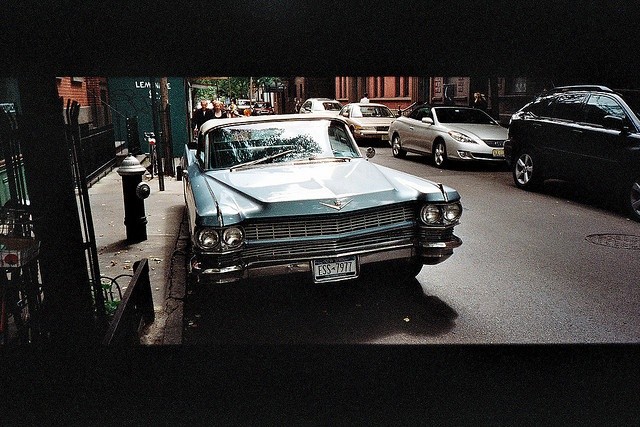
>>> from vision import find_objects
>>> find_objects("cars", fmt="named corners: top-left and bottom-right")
top-left (235, 98), bottom-right (251, 117)
top-left (387, 106), bottom-right (509, 168)
top-left (179, 114), bottom-right (462, 292)
top-left (339, 97), bottom-right (396, 144)
top-left (299, 97), bottom-right (343, 118)
top-left (253, 102), bottom-right (275, 115)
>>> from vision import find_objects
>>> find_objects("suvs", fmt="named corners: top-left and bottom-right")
top-left (502, 84), bottom-right (640, 222)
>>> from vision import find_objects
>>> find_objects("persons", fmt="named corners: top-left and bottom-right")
top-left (191, 99), bottom-right (213, 133)
top-left (212, 100), bottom-right (224, 119)
